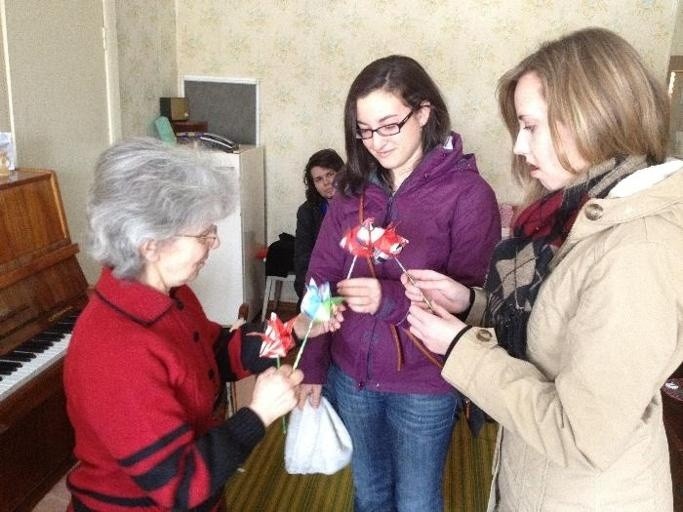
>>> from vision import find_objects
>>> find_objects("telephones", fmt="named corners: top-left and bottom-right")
top-left (196, 131), bottom-right (239, 153)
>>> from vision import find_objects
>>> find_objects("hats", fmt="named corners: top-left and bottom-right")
top-left (285, 394), bottom-right (354, 476)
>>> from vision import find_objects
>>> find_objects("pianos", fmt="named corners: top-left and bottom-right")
top-left (1, 167), bottom-right (94, 511)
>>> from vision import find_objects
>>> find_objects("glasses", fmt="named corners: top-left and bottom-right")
top-left (173, 223), bottom-right (219, 249)
top-left (353, 104), bottom-right (418, 140)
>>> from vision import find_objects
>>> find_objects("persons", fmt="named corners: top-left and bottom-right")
top-left (63, 138), bottom-right (346, 512)
top-left (293, 148), bottom-right (344, 313)
top-left (293, 56), bottom-right (501, 511)
top-left (399, 25), bottom-right (682, 512)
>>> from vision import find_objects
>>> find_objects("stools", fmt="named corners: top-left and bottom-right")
top-left (259, 275), bottom-right (296, 333)
